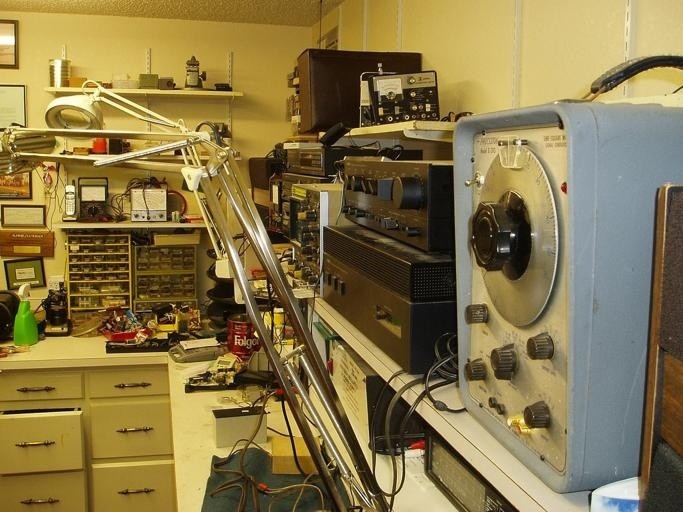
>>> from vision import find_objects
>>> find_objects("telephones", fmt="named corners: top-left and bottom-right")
top-left (62, 179), bottom-right (79, 222)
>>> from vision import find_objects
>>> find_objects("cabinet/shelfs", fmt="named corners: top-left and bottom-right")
top-left (62, 234), bottom-right (200, 338)
top-left (295, 289), bottom-right (591, 512)
top-left (45, 85), bottom-right (245, 230)
top-left (2, 329), bottom-right (176, 512)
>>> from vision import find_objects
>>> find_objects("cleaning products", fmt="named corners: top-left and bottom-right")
top-left (12, 283), bottom-right (39, 346)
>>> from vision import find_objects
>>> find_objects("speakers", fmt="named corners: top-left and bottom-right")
top-left (297, 48), bottom-right (422, 134)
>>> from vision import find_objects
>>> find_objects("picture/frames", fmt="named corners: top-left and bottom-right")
top-left (0, 19), bottom-right (20, 70)
top-left (0, 171), bottom-right (33, 201)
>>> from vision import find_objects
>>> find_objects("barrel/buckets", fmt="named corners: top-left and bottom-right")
top-left (227, 312), bottom-right (262, 358)
